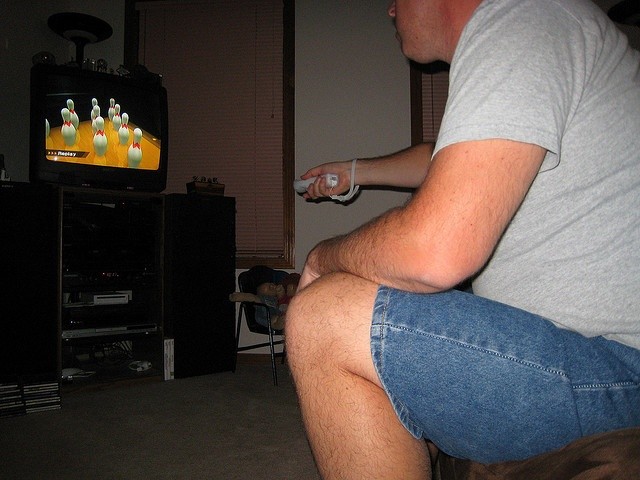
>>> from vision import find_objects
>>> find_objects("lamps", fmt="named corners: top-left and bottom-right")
top-left (47, 12), bottom-right (113, 70)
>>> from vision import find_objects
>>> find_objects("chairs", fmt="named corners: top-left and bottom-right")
top-left (230, 269), bottom-right (298, 388)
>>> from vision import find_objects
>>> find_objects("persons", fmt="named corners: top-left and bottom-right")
top-left (282, 0), bottom-right (640, 480)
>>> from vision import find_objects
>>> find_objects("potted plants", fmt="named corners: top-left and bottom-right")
top-left (186, 176), bottom-right (225, 195)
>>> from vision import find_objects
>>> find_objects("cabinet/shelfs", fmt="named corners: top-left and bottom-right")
top-left (0, 183), bottom-right (58, 381)
top-left (50, 184), bottom-right (164, 380)
top-left (163, 192), bottom-right (237, 378)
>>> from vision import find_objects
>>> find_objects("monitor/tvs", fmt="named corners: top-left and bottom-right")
top-left (29, 65), bottom-right (169, 193)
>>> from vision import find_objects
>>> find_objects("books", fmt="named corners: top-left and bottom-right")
top-left (0, 382), bottom-right (62, 415)
top-left (164, 339), bottom-right (175, 382)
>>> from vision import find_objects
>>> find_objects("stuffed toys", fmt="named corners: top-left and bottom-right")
top-left (275, 273), bottom-right (302, 313)
top-left (229, 266), bottom-right (285, 330)
